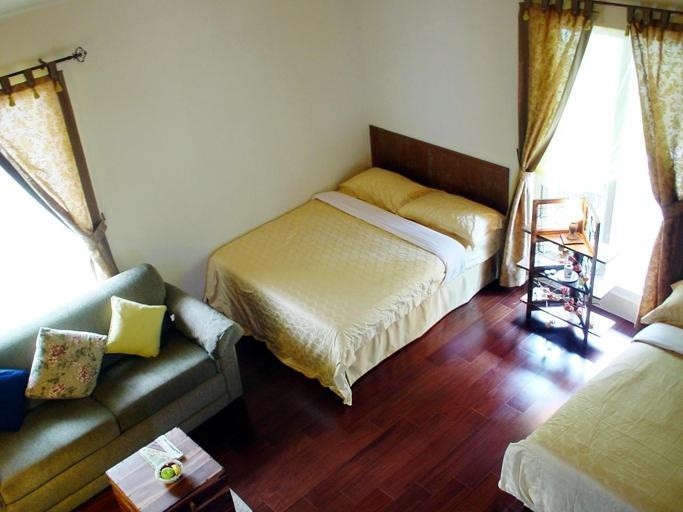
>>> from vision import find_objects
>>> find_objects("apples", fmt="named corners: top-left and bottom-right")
top-left (172, 464), bottom-right (181, 475)
top-left (160, 466), bottom-right (174, 479)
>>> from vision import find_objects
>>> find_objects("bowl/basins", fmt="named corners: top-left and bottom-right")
top-left (154, 459), bottom-right (183, 485)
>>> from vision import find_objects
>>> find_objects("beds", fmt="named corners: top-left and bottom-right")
top-left (497, 276), bottom-right (683, 512)
top-left (200, 125), bottom-right (509, 405)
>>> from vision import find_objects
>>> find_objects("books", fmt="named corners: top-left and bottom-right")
top-left (139, 435), bottom-right (183, 470)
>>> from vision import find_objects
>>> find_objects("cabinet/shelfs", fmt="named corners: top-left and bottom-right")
top-left (526, 196), bottom-right (601, 357)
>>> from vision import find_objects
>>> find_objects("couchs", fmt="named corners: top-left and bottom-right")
top-left (1, 263), bottom-right (245, 512)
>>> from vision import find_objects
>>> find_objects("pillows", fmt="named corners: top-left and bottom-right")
top-left (104, 294), bottom-right (167, 358)
top-left (339, 168), bottom-right (440, 214)
top-left (640, 279), bottom-right (683, 325)
top-left (397, 189), bottom-right (506, 251)
top-left (0, 368), bottom-right (31, 433)
top-left (25, 327), bottom-right (106, 400)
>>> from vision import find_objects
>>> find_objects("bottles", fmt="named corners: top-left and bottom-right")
top-left (564, 264), bottom-right (573, 278)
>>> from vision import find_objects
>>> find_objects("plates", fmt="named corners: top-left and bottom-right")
top-left (556, 269), bottom-right (579, 283)
